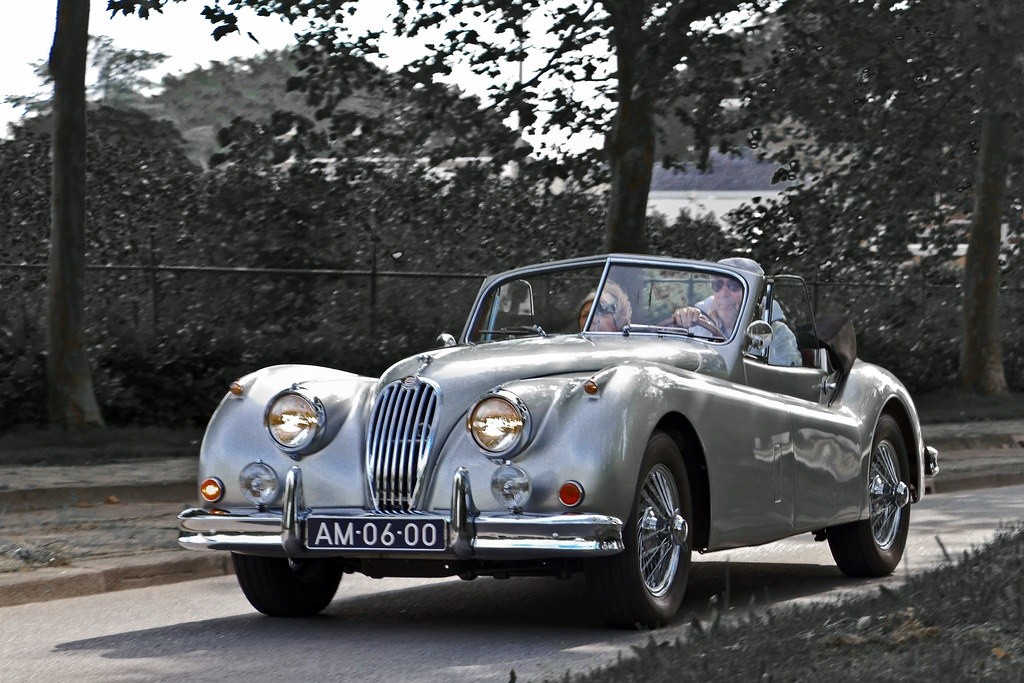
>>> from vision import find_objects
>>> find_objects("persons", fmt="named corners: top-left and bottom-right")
top-left (579, 280), bottom-right (634, 331)
top-left (670, 257), bottom-right (803, 367)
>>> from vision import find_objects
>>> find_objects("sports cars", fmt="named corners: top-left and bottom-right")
top-left (174, 248), bottom-right (944, 634)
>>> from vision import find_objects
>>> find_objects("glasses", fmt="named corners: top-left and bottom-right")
top-left (712, 279), bottom-right (741, 292)
